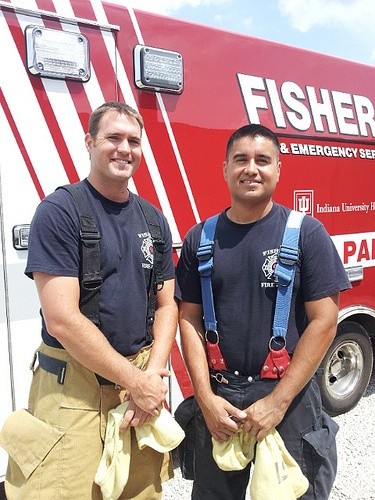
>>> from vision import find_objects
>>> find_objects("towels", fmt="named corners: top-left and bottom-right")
top-left (95, 399), bottom-right (189, 496)
top-left (210, 415), bottom-right (310, 496)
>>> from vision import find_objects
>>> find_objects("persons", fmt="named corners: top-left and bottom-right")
top-left (174, 123), bottom-right (352, 500)
top-left (0, 101), bottom-right (186, 500)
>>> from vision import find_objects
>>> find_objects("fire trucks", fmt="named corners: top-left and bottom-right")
top-left (0, 0), bottom-right (375, 480)
